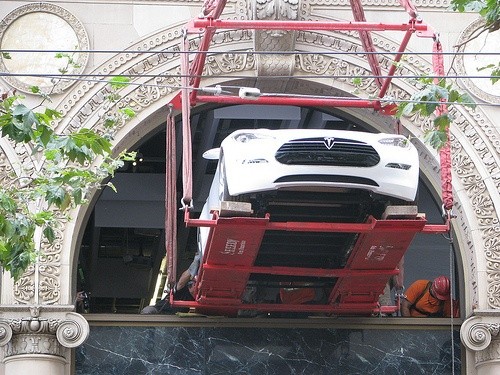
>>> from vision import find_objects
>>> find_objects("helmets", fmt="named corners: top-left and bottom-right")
top-left (432, 274), bottom-right (452, 300)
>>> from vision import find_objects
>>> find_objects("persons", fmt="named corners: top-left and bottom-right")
top-left (142, 250), bottom-right (202, 315)
top-left (400, 276), bottom-right (459, 318)
top-left (370, 253), bottom-right (404, 317)
top-left (279, 278), bottom-right (327, 318)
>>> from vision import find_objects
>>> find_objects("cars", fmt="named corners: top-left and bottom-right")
top-left (191, 127), bottom-right (419, 277)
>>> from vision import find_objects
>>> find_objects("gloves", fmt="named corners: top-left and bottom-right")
top-left (188, 254), bottom-right (201, 278)
